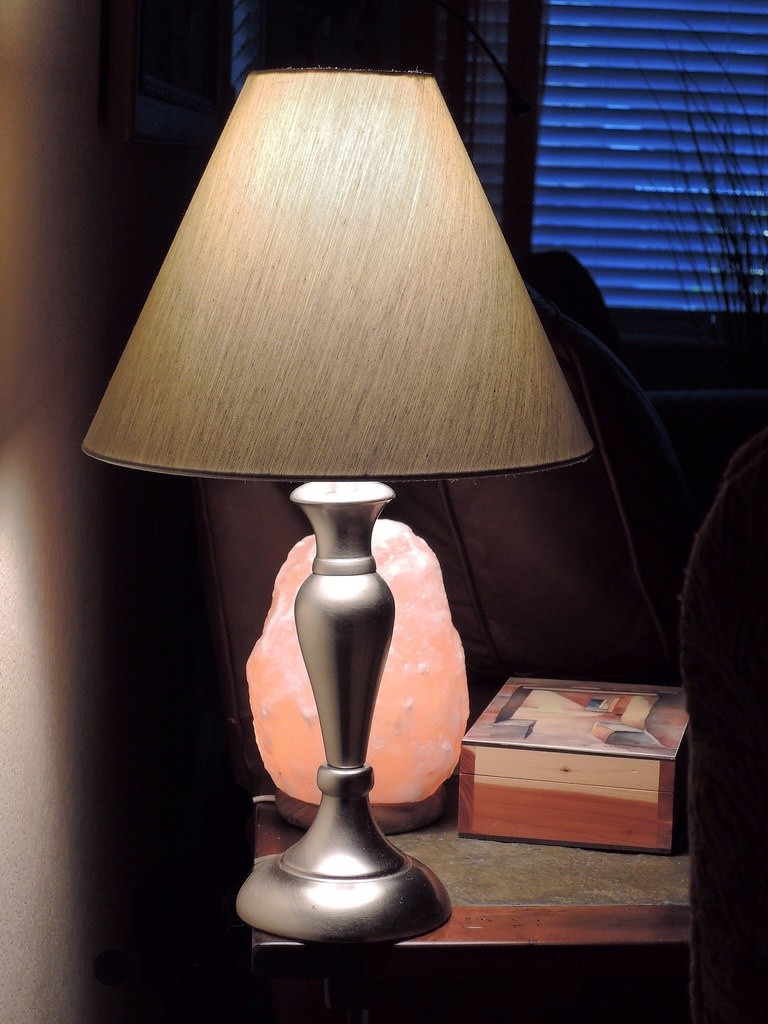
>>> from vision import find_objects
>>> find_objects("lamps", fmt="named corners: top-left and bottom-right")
top-left (80, 67), bottom-right (595, 944)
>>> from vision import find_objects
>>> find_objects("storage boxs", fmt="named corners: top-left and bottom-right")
top-left (457, 676), bottom-right (690, 854)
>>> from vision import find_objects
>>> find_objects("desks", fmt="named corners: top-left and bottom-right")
top-left (243, 789), bottom-right (697, 1024)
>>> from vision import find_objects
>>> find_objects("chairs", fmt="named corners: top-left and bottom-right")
top-left (189, 259), bottom-right (767, 1024)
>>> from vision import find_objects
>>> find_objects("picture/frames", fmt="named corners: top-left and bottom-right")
top-left (110, 0), bottom-right (233, 144)
